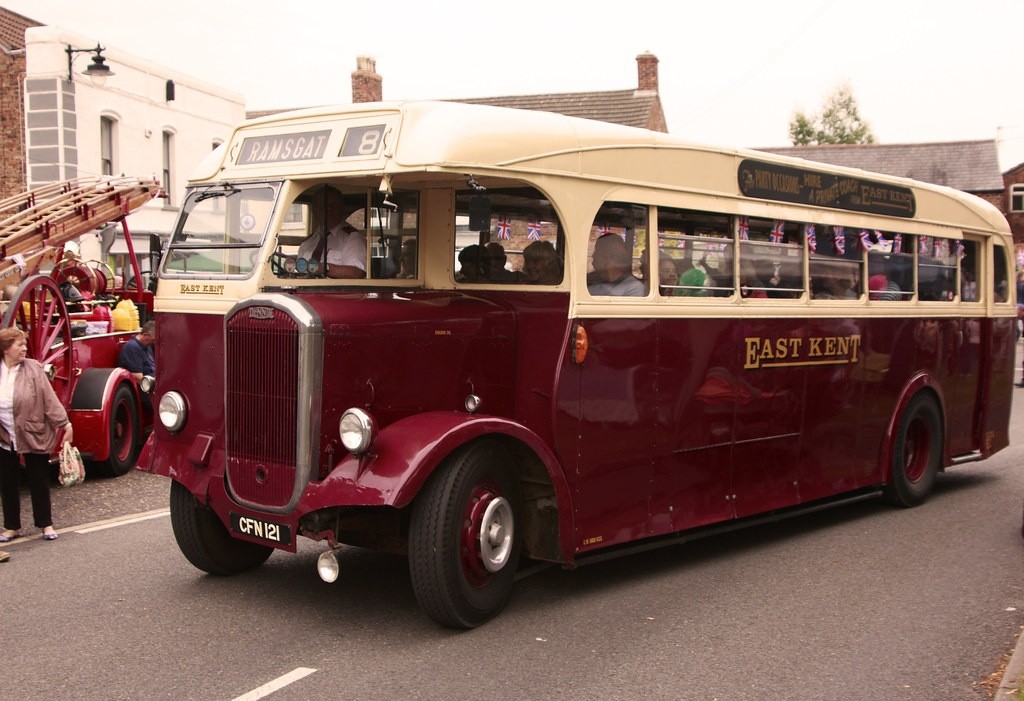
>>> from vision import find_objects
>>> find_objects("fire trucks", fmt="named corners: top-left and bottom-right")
top-left (0, 173), bottom-right (170, 476)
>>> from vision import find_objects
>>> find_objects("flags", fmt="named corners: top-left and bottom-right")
top-left (598, 226), bottom-right (636, 246)
top-left (800, 224), bottom-right (817, 256)
top-left (658, 239), bottom-right (665, 252)
top-left (496, 216), bottom-right (511, 241)
top-left (677, 232), bottom-right (685, 248)
top-left (859, 230), bottom-right (965, 257)
top-left (833, 226), bottom-right (846, 254)
top-left (527, 220), bottom-right (541, 241)
top-left (768, 219), bottom-right (785, 243)
top-left (705, 234), bottom-right (729, 256)
top-left (739, 216), bottom-right (749, 240)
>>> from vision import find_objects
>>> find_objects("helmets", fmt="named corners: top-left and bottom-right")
top-left (87, 299), bottom-right (140, 332)
top-left (59, 282), bottom-right (87, 302)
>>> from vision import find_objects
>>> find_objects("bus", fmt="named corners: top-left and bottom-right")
top-left (135, 98), bottom-right (1018, 632)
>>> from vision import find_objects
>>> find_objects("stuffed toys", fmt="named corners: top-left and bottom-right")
top-left (869, 274), bottom-right (887, 297)
top-left (674, 269), bottom-right (708, 297)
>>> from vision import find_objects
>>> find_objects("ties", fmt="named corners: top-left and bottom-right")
top-left (311, 234), bottom-right (326, 263)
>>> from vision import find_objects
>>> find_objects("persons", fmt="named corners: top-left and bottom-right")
top-left (1016, 272), bottom-right (1024, 337)
top-left (588, 233), bottom-right (679, 296)
top-left (297, 187), bottom-right (367, 279)
top-left (458, 240), bottom-right (558, 284)
top-left (809, 255), bottom-right (902, 300)
top-left (699, 243), bottom-right (803, 298)
top-left (118, 320), bottom-right (155, 385)
top-left (0, 328), bottom-right (73, 542)
top-left (396, 239), bottom-right (416, 279)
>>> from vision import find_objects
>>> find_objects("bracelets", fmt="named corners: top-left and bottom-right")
top-left (327, 263), bottom-right (330, 272)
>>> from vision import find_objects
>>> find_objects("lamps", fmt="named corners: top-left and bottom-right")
top-left (65, 42), bottom-right (116, 84)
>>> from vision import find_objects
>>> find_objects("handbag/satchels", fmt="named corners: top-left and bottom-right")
top-left (57, 441), bottom-right (86, 487)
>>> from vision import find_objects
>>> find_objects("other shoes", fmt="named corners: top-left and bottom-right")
top-left (0, 529), bottom-right (22, 541)
top-left (0, 551), bottom-right (10, 561)
top-left (42, 528), bottom-right (58, 540)
